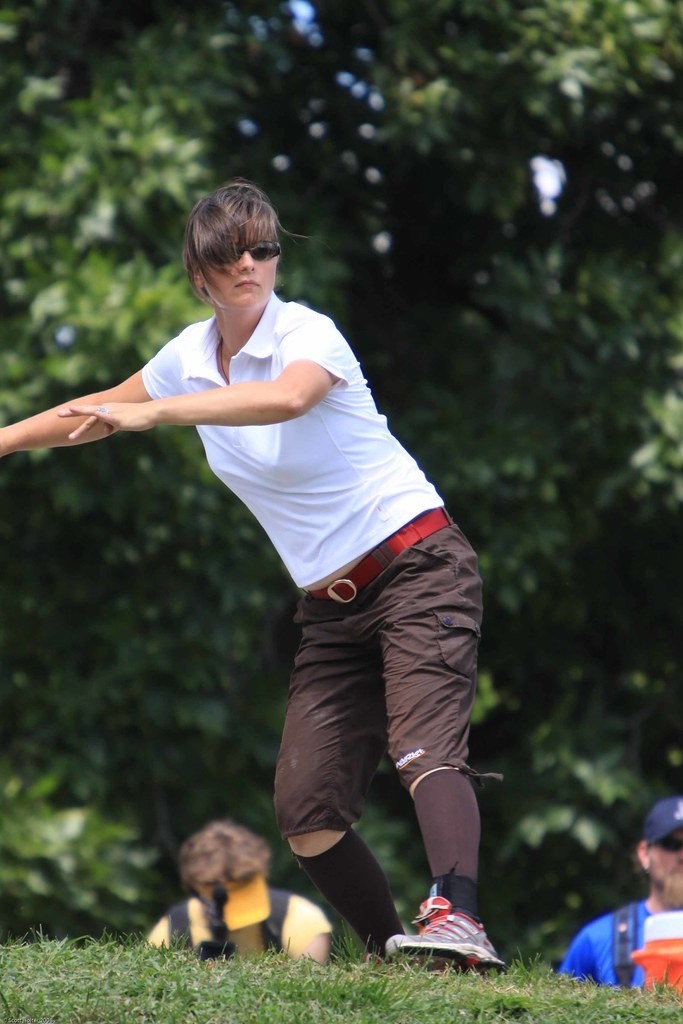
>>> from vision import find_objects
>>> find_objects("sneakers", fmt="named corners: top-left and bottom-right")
top-left (386, 896), bottom-right (505, 972)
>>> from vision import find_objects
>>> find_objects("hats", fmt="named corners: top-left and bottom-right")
top-left (193, 870), bottom-right (271, 929)
top-left (643, 797), bottom-right (683, 844)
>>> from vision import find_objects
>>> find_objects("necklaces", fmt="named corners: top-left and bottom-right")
top-left (221, 342), bottom-right (229, 383)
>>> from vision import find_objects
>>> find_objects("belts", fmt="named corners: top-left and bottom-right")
top-left (309, 509), bottom-right (450, 604)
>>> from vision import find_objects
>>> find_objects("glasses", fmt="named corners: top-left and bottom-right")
top-left (658, 839), bottom-right (683, 851)
top-left (215, 242), bottom-right (281, 263)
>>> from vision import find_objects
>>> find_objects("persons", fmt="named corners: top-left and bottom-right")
top-left (0, 183), bottom-right (507, 976)
top-left (147, 822), bottom-right (332, 964)
top-left (559, 796), bottom-right (683, 993)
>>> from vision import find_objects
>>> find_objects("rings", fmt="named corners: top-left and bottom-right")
top-left (97, 407), bottom-right (108, 413)
